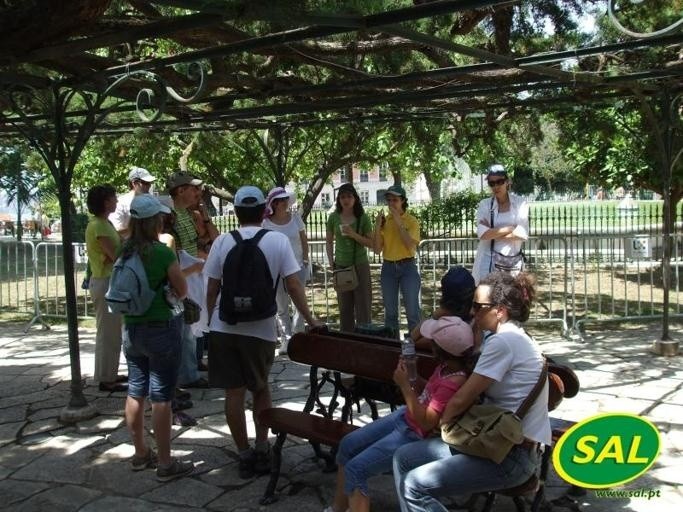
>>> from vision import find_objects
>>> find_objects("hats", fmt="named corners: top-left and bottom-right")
top-left (485, 164), bottom-right (507, 180)
top-left (267, 187), bottom-right (295, 214)
top-left (130, 194), bottom-right (171, 219)
top-left (234, 187), bottom-right (266, 207)
top-left (420, 317), bottom-right (474, 356)
top-left (129, 168), bottom-right (156, 182)
top-left (338, 184), bottom-right (355, 193)
top-left (166, 171), bottom-right (203, 191)
top-left (439, 267), bottom-right (477, 292)
top-left (384, 185), bottom-right (406, 198)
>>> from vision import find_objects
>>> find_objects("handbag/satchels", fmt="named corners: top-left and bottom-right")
top-left (489, 250), bottom-right (526, 277)
top-left (352, 324), bottom-right (407, 399)
top-left (82, 262), bottom-right (92, 289)
top-left (441, 404), bottom-right (524, 464)
top-left (184, 297), bottom-right (202, 324)
top-left (333, 265), bottom-right (359, 291)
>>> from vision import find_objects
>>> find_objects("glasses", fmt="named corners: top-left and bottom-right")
top-left (487, 178), bottom-right (507, 187)
top-left (472, 301), bottom-right (495, 313)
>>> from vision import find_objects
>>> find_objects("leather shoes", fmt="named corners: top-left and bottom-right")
top-left (99, 383), bottom-right (129, 391)
top-left (115, 375), bottom-right (128, 381)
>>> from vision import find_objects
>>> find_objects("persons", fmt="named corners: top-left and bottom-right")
top-left (410, 266), bottom-right (481, 361)
top-left (326, 183), bottom-right (373, 332)
top-left (472, 165), bottom-right (530, 287)
top-left (201, 186), bottom-right (324, 478)
top-left (372, 186), bottom-right (421, 338)
top-left (391, 273), bottom-right (551, 512)
top-left (114, 193), bottom-right (194, 483)
top-left (84, 168), bottom-right (309, 392)
top-left (322, 315), bottom-right (473, 512)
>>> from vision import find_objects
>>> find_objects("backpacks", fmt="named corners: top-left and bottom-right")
top-left (104, 241), bottom-right (168, 316)
top-left (219, 229), bottom-right (280, 325)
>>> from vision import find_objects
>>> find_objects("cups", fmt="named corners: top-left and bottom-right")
top-left (339, 224), bottom-right (350, 236)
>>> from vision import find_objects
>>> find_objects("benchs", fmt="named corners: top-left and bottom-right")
top-left (306, 325), bottom-right (587, 497)
top-left (253, 325), bottom-right (564, 511)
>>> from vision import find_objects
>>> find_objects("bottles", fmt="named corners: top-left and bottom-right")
top-left (401, 331), bottom-right (421, 383)
top-left (162, 286), bottom-right (185, 316)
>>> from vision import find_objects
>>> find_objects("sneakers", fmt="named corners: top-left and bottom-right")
top-left (277, 335), bottom-right (291, 354)
top-left (172, 398), bottom-right (193, 410)
top-left (198, 360), bottom-right (208, 371)
top-left (253, 441), bottom-right (275, 473)
top-left (239, 446), bottom-right (255, 478)
top-left (132, 447), bottom-right (156, 469)
top-left (175, 388), bottom-right (190, 399)
top-left (156, 459), bottom-right (193, 482)
top-left (179, 377), bottom-right (209, 388)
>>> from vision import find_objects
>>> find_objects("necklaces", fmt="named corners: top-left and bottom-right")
top-left (498, 199), bottom-right (507, 204)
